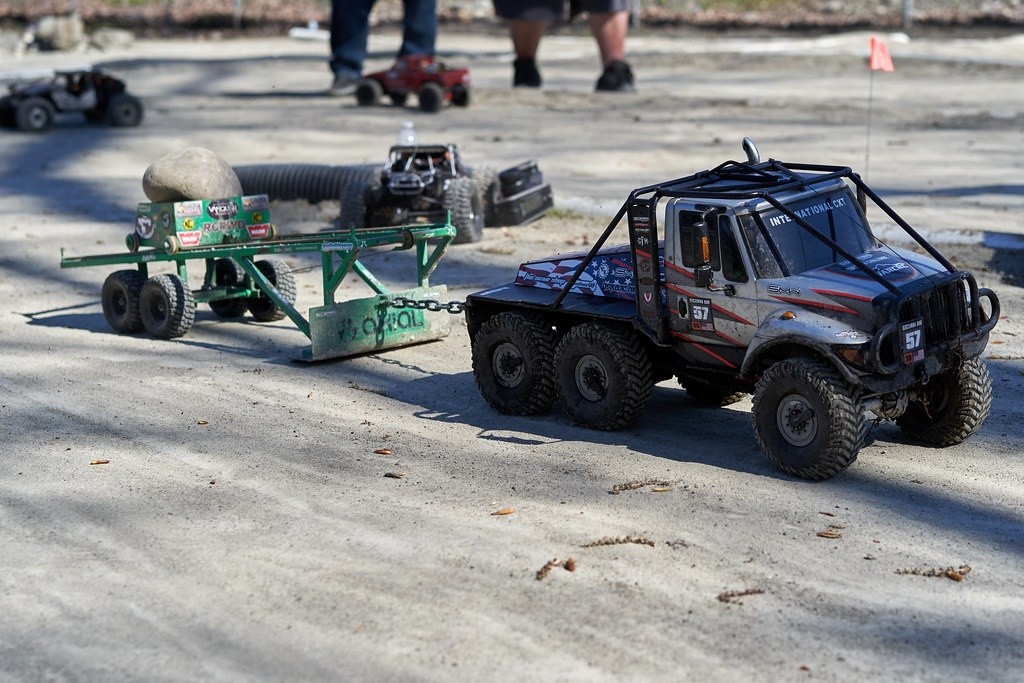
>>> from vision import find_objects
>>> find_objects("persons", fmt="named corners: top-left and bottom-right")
top-left (493, 0), bottom-right (635, 91)
top-left (329, 0), bottom-right (436, 95)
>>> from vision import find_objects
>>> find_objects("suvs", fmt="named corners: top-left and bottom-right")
top-left (459, 136), bottom-right (1002, 481)
top-left (363, 142), bottom-right (485, 245)
top-left (0, 63), bottom-right (144, 134)
top-left (350, 53), bottom-right (474, 112)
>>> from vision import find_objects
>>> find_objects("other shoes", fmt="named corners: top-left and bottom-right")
top-left (329, 77), bottom-right (358, 94)
top-left (512, 55), bottom-right (541, 86)
top-left (597, 61), bottom-right (635, 91)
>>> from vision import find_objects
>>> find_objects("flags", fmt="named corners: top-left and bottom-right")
top-left (869, 38), bottom-right (894, 72)
